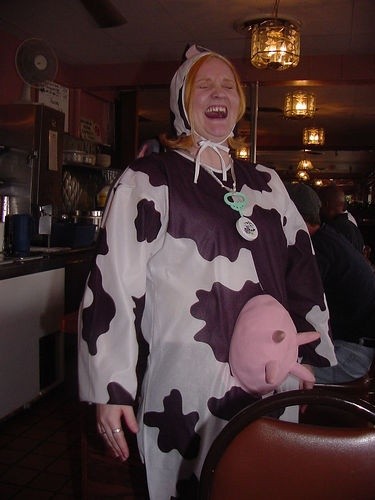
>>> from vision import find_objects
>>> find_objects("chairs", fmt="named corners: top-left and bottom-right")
top-left (199, 389), bottom-right (375, 500)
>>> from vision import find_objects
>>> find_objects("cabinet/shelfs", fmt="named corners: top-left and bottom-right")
top-left (63, 162), bottom-right (125, 240)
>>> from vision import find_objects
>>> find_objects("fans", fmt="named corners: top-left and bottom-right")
top-left (12, 37), bottom-right (58, 105)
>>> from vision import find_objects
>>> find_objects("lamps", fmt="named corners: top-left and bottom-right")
top-left (297, 148), bottom-right (313, 170)
top-left (251, 19), bottom-right (300, 72)
top-left (282, 90), bottom-right (316, 120)
top-left (235, 144), bottom-right (250, 159)
top-left (303, 127), bottom-right (324, 145)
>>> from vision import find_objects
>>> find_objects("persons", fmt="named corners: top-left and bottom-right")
top-left (287, 183), bottom-right (375, 340)
top-left (77, 45), bottom-right (340, 500)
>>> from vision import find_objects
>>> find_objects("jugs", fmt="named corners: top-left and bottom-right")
top-left (4, 212), bottom-right (35, 258)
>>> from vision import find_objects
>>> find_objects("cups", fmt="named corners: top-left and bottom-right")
top-left (97, 153), bottom-right (111, 166)
top-left (63, 149), bottom-right (96, 165)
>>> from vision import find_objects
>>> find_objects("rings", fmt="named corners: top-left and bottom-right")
top-left (113, 429), bottom-right (122, 433)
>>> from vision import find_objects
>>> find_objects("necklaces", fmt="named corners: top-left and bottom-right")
top-left (188, 153), bottom-right (258, 242)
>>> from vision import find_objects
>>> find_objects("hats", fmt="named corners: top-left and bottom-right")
top-left (283, 182), bottom-right (321, 214)
top-left (169, 43), bottom-right (237, 183)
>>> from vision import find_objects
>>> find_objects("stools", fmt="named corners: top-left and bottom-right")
top-left (312, 371), bottom-right (369, 428)
top-left (81, 407), bottom-right (136, 500)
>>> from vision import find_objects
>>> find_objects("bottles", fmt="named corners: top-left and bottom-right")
top-left (90, 209), bottom-right (102, 233)
